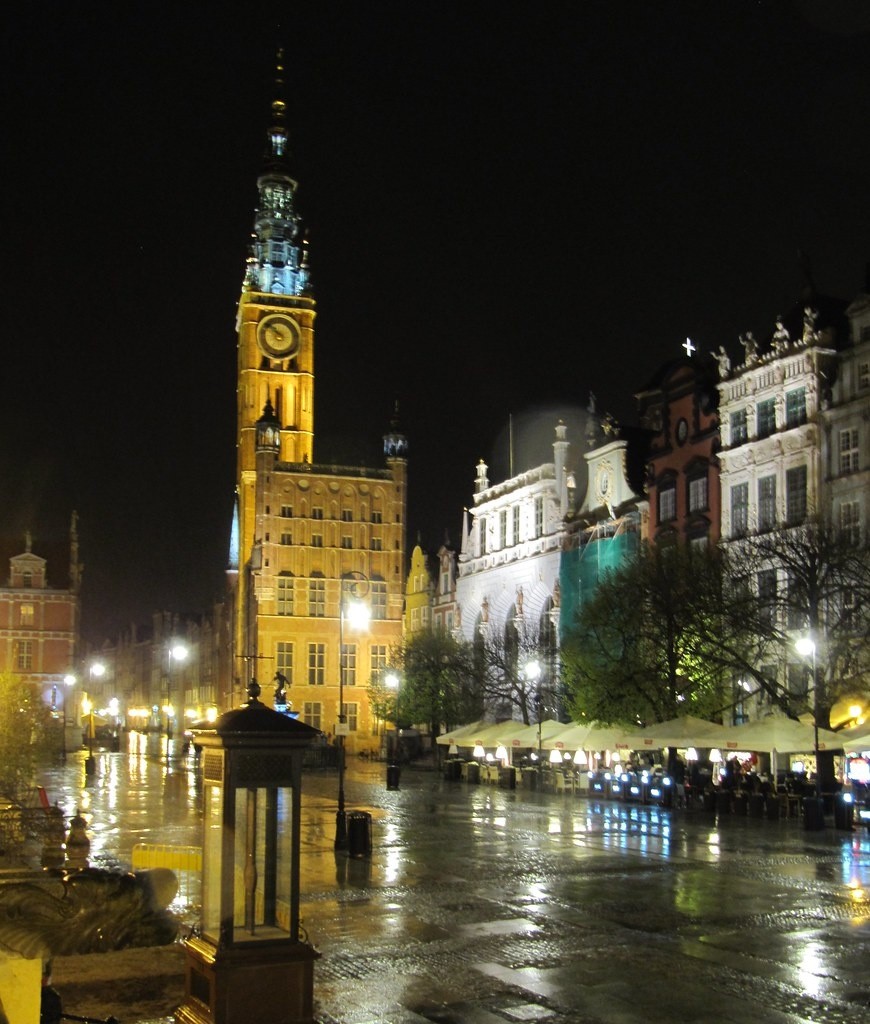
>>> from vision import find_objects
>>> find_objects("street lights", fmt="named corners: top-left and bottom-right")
top-left (386, 671), bottom-right (399, 736)
top-left (166, 639), bottom-right (184, 759)
top-left (63, 673), bottom-right (74, 761)
top-left (89, 658), bottom-right (101, 757)
top-left (531, 657), bottom-right (543, 770)
top-left (800, 627), bottom-right (820, 797)
top-left (334, 570), bottom-right (369, 848)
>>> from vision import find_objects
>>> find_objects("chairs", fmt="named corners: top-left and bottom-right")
top-left (463, 763), bottom-right (802, 822)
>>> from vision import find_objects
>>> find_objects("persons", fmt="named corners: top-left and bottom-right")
top-left (803, 306), bottom-right (823, 345)
top-left (598, 756), bottom-right (862, 818)
top-left (739, 332), bottom-right (758, 361)
top-left (273, 672), bottom-right (291, 697)
top-left (246, 678), bottom-right (261, 700)
top-left (770, 320), bottom-right (789, 349)
top-left (711, 346), bottom-right (731, 376)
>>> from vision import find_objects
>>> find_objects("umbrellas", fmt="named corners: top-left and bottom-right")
top-left (437, 704), bottom-right (870, 790)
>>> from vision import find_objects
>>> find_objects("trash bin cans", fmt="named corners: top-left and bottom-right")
top-left (345, 811), bottom-right (372, 855)
top-left (835, 794), bottom-right (854, 828)
top-left (467, 761), bottom-right (480, 782)
top-left (386, 765), bottom-right (402, 789)
top-left (803, 797), bottom-right (824, 831)
top-left (85, 756), bottom-right (96, 774)
top-left (500, 765), bottom-right (515, 789)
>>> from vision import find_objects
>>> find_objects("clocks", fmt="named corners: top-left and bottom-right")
top-left (262, 317), bottom-right (300, 355)
